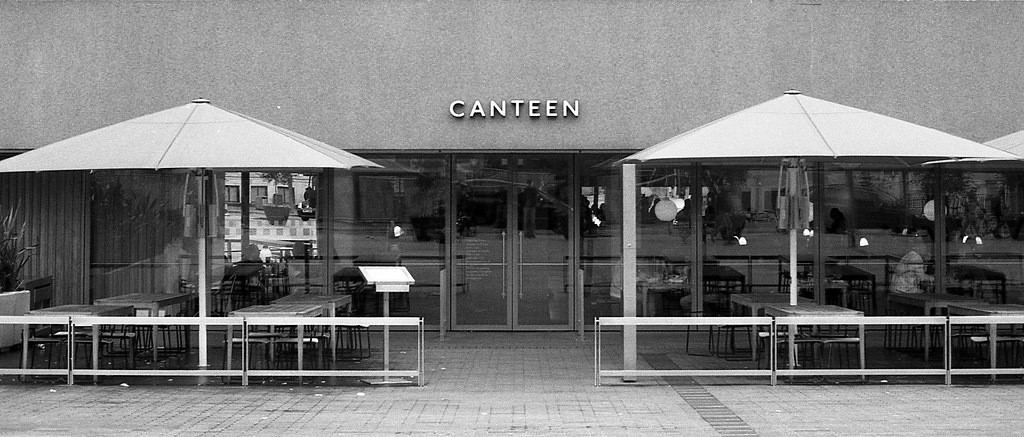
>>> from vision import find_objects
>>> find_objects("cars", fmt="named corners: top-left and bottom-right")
top-left (803, 184), bottom-right (926, 235)
top-left (456, 177), bottom-right (581, 237)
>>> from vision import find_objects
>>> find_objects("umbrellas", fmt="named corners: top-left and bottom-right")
top-left (2, 94), bottom-right (384, 369)
top-left (918, 129), bottom-right (1024, 174)
top-left (607, 84), bottom-right (1023, 374)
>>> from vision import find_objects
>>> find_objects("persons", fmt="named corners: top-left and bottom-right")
top-left (890, 240), bottom-right (934, 294)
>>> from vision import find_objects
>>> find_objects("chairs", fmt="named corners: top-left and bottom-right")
top-left (584, 257), bottom-right (1023, 385)
top-left (1, 253), bottom-right (415, 388)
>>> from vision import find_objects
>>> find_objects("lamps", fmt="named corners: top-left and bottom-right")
top-left (654, 167), bottom-right (678, 222)
top-left (384, 219), bottom-right (405, 252)
